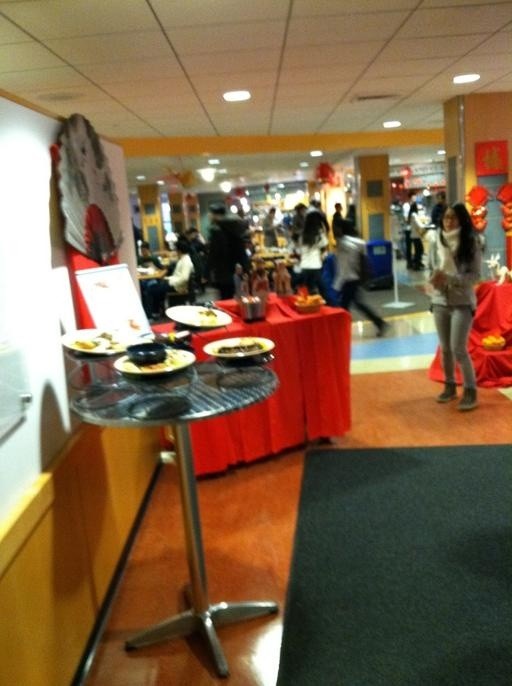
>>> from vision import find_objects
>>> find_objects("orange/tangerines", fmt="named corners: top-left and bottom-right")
top-left (296, 293), bottom-right (323, 306)
top-left (481, 335), bottom-right (506, 348)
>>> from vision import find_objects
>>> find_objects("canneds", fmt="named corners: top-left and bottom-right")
top-left (240, 295), bottom-right (265, 321)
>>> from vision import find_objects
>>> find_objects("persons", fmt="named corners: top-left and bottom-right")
top-left (425, 201), bottom-right (482, 411)
top-left (402, 191), bottom-right (426, 271)
top-left (288, 200), bottom-right (389, 338)
top-left (136, 206), bottom-right (252, 322)
top-left (261, 207), bottom-right (279, 282)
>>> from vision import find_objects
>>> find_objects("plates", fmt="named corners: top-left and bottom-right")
top-left (164, 305), bottom-right (233, 329)
top-left (60, 328), bottom-right (140, 355)
top-left (113, 348), bottom-right (197, 377)
top-left (203, 337), bottom-right (276, 359)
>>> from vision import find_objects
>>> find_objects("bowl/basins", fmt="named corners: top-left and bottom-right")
top-left (126, 342), bottom-right (166, 366)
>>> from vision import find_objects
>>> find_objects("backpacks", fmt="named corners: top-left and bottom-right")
top-left (358, 255), bottom-right (371, 284)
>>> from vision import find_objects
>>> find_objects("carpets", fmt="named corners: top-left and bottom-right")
top-left (277, 441), bottom-right (511, 686)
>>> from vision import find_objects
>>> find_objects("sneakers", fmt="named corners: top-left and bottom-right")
top-left (457, 388), bottom-right (478, 411)
top-left (376, 323), bottom-right (391, 337)
top-left (437, 382), bottom-right (458, 403)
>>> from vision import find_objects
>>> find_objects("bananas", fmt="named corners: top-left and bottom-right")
top-left (76, 337), bottom-right (112, 350)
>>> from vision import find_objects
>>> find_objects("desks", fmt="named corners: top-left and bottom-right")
top-left (136, 232), bottom-right (300, 316)
top-left (150, 294), bottom-right (351, 480)
top-left (68, 362), bottom-right (278, 677)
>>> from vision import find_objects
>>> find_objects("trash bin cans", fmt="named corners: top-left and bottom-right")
top-left (366, 239), bottom-right (394, 291)
